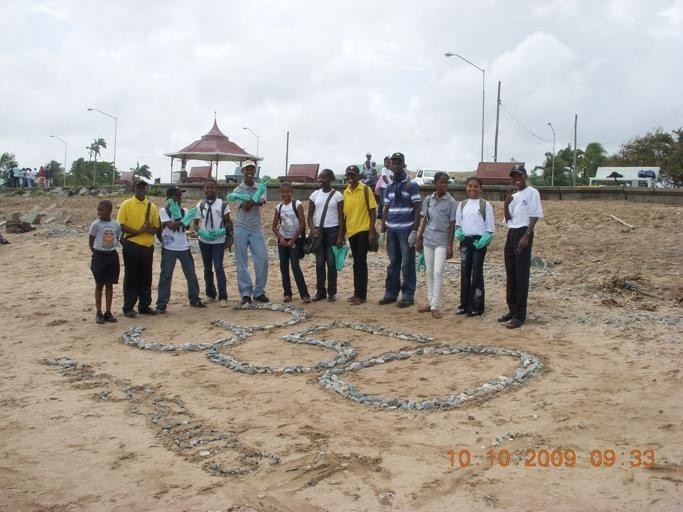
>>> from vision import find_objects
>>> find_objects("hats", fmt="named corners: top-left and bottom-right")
top-left (346, 165), bottom-right (359, 175)
top-left (509, 165), bottom-right (526, 176)
top-left (241, 159), bottom-right (256, 170)
top-left (133, 179), bottom-right (148, 186)
top-left (390, 152), bottom-right (404, 160)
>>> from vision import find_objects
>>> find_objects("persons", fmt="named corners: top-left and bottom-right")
top-left (306, 168), bottom-right (345, 303)
top-left (359, 153), bottom-right (380, 185)
top-left (496, 163), bottom-right (545, 330)
top-left (343, 165), bottom-right (377, 306)
top-left (416, 170), bottom-right (457, 320)
top-left (456, 176), bottom-right (496, 316)
top-left (379, 152), bottom-right (423, 309)
top-left (377, 155), bottom-right (393, 219)
top-left (5, 162), bottom-right (54, 190)
top-left (360, 161), bottom-right (378, 187)
top-left (116, 178), bottom-right (161, 318)
top-left (271, 182), bottom-right (312, 303)
top-left (232, 159), bottom-right (270, 307)
top-left (194, 179), bottom-right (234, 308)
top-left (158, 186), bottom-right (206, 314)
top-left (88, 200), bottom-right (121, 325)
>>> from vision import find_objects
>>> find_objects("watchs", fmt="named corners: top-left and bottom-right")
top-left (526, 230), bottom-right (532, 237)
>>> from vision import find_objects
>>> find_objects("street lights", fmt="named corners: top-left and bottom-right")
top-left (444, 52), bottom-right (484, 163)
top-left (50, 135), bottom-right (68, 187)
top-left (242, 127), bottom-right (260, 158)
top-left (86, 108), bottom-right (118, 185)
top-left (547, 121), bottom-right (555, 187)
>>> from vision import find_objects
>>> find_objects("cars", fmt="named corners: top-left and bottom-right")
top-left (409, 169), bottom-right (455, 186)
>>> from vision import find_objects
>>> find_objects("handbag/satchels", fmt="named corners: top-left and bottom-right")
top-left (121, 232), bottom-right (125, 244)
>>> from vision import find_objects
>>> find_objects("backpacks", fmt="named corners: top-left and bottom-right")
top-left (157, 206), bottom-right (185, 242)
